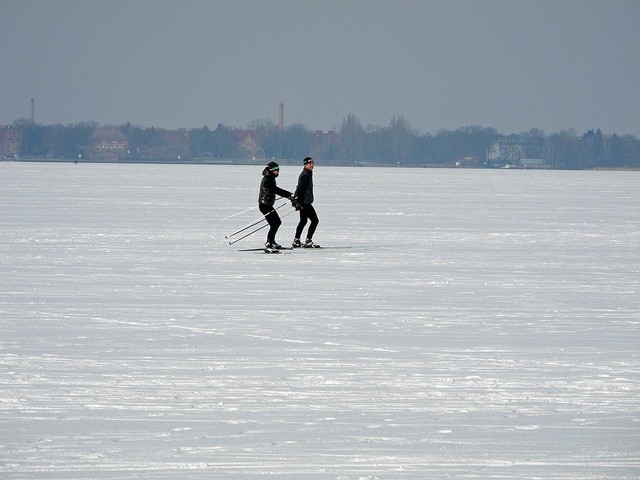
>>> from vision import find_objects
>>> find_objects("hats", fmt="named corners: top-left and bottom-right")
top-left (303, 157), bottom-right (314, 165)
top-left (267, 161), bottom-right (279, 171)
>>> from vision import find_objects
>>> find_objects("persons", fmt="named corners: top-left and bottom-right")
top-left (258, 162), bottom-right (295, 254)
top-left (292, 157), bottom-right (320, 248)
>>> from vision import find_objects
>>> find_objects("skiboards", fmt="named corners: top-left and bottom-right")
top-left (296, 243), bottom-right (352, 249)
top-left (237, 246), bottom-right (294, 256)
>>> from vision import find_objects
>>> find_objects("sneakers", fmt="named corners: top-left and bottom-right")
top-left (270, 240), bottom-right (282, 248)
top-left (292, 238), bottom-right (305, 248)
top-left (304, 240), bottom-right (319, 247)
top-left (265, 241), bottom-right (279, 254)
